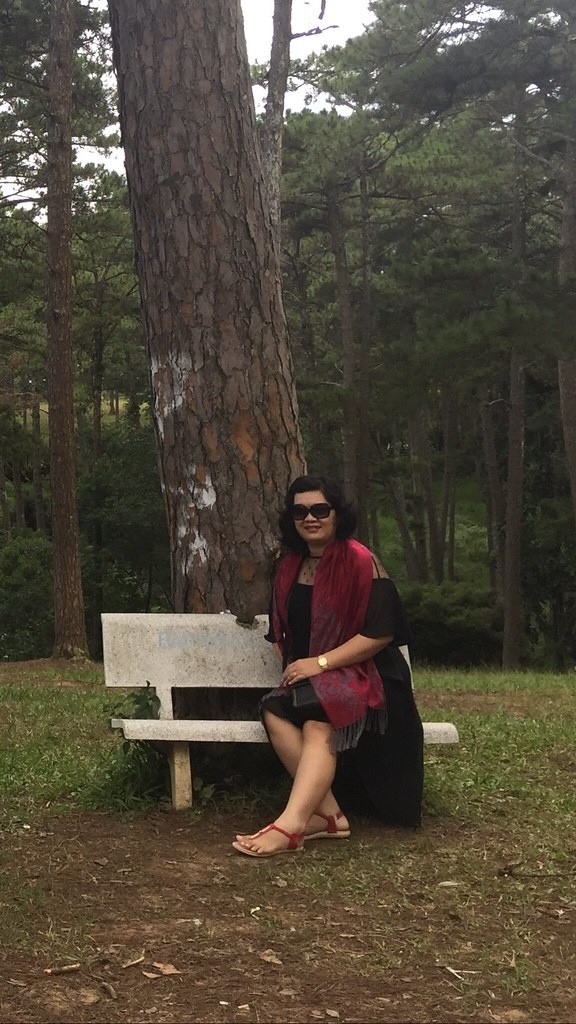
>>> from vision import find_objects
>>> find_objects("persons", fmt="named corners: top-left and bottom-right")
top-left (232, 475), bottom-right (402, 858)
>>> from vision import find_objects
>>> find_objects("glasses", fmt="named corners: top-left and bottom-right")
top-left (290, 503), bottom-right (334, 520)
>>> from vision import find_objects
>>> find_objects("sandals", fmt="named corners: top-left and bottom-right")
top-left (304, 810), bottom-right (350, 840)
top-left (232, 823), bottom-right (304, 857)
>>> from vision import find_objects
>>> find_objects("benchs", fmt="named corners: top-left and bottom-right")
top-left (101, 610), bottom-right (459, 809)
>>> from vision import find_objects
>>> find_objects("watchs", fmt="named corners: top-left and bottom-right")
top-left (318, 655), bottom-right (330, 672)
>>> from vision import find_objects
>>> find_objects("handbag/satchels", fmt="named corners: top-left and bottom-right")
top-left (289, 681), bottom-right (330, 723)
